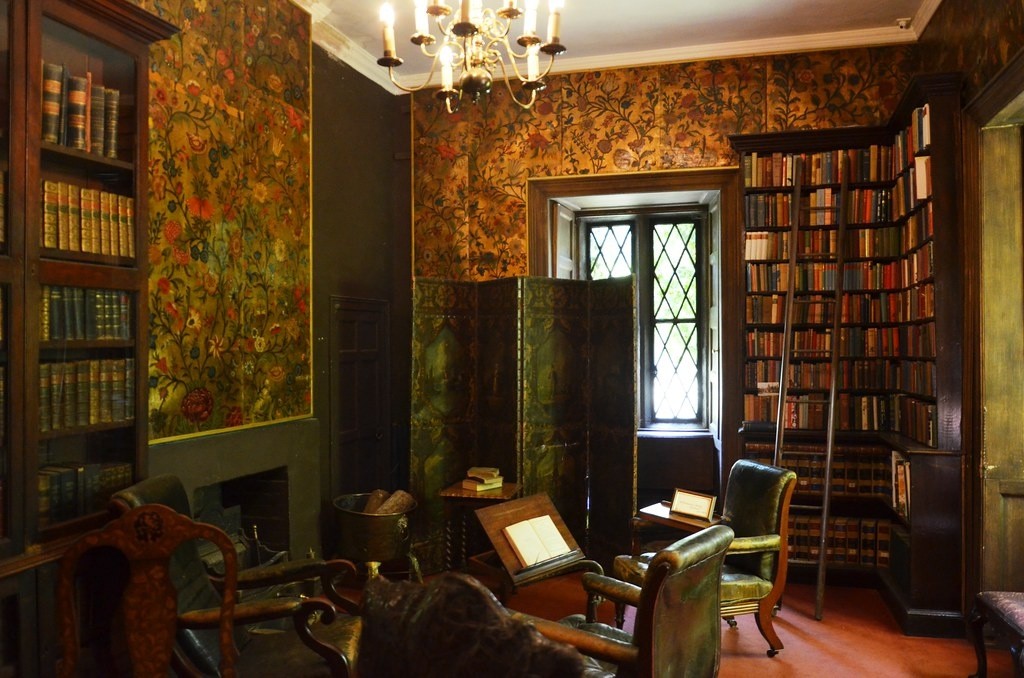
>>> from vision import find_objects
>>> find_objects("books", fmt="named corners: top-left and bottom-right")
top-left (463, 467), bottom-right (503, 491)
top-left (38, 358), bottom-right (135, 430)
top-left (41, 181), bottom-right (134, 257)
top-left (503, 513), bottom-right (570, 569)
top-left (746, 102), bottom-right (937, 448)
top-left (35, 464), bottom-right (133, 525)
top-left (745, 442), bottom-right (911, 569)
top-left (38, 282), bottom-right (132, 339)
top-left (39, 59), bottom-right (120, 159)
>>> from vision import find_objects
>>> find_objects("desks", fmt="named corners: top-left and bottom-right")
top-left (439, 479), bottom-right (523, 575)
top-left (628, 501), bottom-right (723, 556)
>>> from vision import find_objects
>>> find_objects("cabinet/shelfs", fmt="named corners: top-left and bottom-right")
top-left (726, 69), bottom-right (975, 637)
top-left (0, 0), bottom-right (180, 678)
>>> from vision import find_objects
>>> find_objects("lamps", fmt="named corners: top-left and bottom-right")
top-left (377, 1), bottom-right (566, 116)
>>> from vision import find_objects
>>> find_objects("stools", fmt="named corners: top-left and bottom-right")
top-left (968, 592), bottom-right (1024, 678)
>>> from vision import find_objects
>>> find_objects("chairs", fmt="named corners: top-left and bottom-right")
top-left (108, 472), bottom-right (361, 678)
top-left (612, 459), bottom-right (797, 659)
top-left (60, 503), bottom-right (240, 678)
top-left (501, 525), bottom-right (734, 678)
top-left (361, 570), bottom-right (587, 678)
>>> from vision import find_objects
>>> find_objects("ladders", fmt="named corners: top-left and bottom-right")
top-left (771, 151), bottom-right (853, 625)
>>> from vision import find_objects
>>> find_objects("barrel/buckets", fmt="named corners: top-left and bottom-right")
top-left (333, 493), bottom-right (418, 572)
